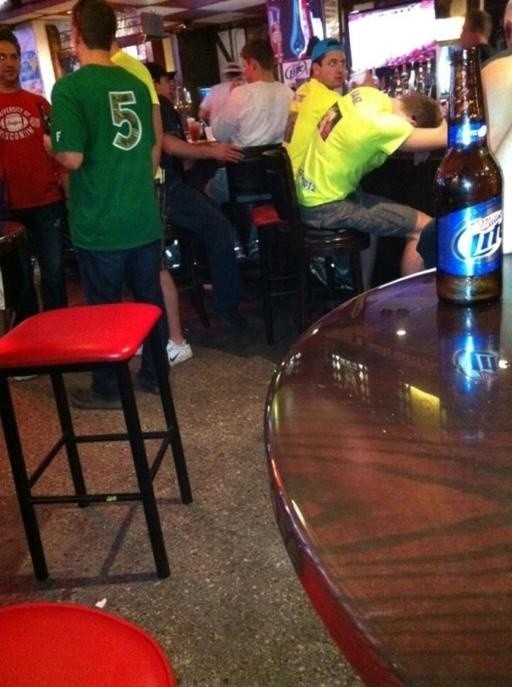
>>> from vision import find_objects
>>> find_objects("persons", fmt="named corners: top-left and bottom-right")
top-left (1, 30), bottom-right (67, 381)
top-left (463, 12), bottom-right (493, 62)
top-left (44, 3), bottom-right (191, 410)
top-left (197, 38), bottom-right (446, 290)
top-left (142, 60), bottom-right (264, 322)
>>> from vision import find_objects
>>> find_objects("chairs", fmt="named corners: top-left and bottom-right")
top-left (162, 225), bottom-right (213, 329)
top-left (223, 145), bottom-right (370, 345)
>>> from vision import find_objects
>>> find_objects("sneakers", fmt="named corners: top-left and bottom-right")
top-left (232, 236), bottom-right (265, 278)
top-left (128, 368), bottom-right (163, 396)
top-left (70, 377), bottom-right (123, 409)
top-left (206, 302), bottom-right (249, 333)
top-left (165, 337), bottom-right (195, 370)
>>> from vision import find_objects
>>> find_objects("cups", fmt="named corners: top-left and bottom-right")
top-left (188, 122), bottom-right (203, 144)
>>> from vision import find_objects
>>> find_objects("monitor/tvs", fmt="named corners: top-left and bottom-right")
top-left (344, 0), bottom-right (439, 78)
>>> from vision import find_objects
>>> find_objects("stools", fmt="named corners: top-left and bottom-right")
top-left (1, 602), bottom-right (179, 686)
top-left (1, 302), bottom-right (196, 582)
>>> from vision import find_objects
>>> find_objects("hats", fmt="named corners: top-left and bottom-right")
top-left (311, 38), bottom-right (345, 63)
top-left (221, 62), bottom-right (243, 73)
top-left (144, 61), bottom-right (178, 81)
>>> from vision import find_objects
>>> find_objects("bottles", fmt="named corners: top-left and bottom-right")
top-left (373, 58), bottom-right (436, 101)
top-left (432, 46), bottom-right (503, 305)
top-left (34, 100), bottom-right (54, 137)
